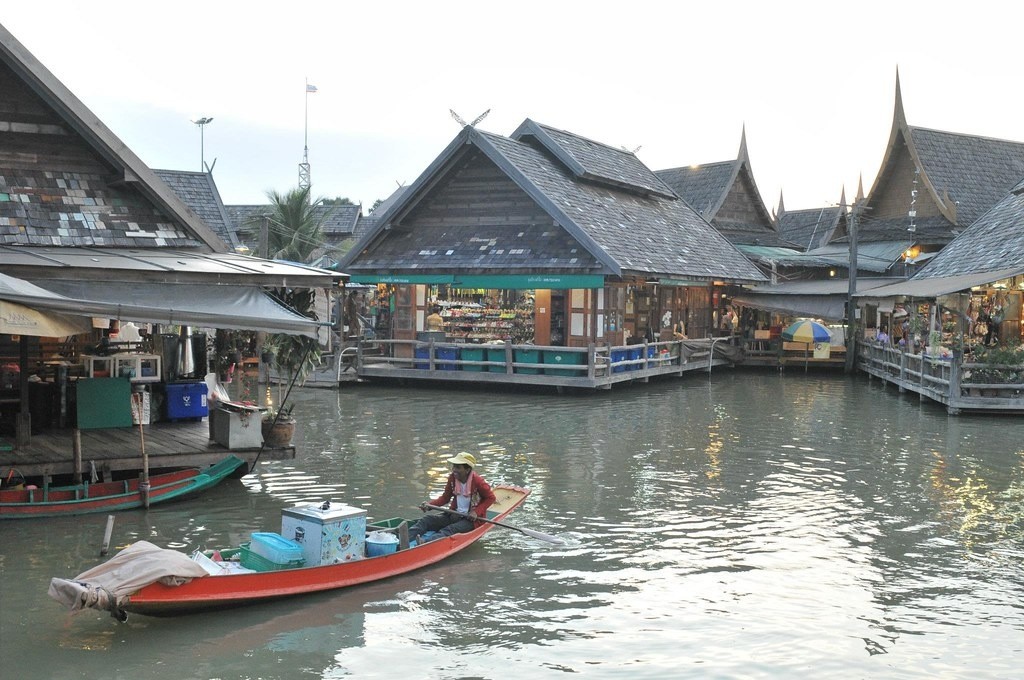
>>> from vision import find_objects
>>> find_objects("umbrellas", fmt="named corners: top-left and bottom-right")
top-left (0, 301), bottom-right (92, 337)
top-left (783, 321), bottom-right (833, 361)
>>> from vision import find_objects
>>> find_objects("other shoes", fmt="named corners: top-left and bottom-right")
top-left (416, 534), bottom-right (424, 544)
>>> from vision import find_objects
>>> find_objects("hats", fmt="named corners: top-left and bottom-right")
top-left (447, 452), bottom-right (476, 469)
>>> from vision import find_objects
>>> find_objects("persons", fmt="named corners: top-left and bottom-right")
top-left (345, 291), bottom-right (360, 337)
top-left (902, 314), bottom-right (910, 336)
top-left (899, 331), bottom-right (911, 346)
top-left (426, 307), bottom-right (443, 330)
top-left (405, 452), bottom-right (496, 543)
top-left (876, 326), bottom-right (889, 343)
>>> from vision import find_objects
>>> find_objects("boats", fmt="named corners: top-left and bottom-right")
top-left (0, 452), bottom-right (245, 520)
top-left (51, 484), bottom-right (532, 615)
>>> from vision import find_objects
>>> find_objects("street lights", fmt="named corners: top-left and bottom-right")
top-left (190, 117), bottom-right (214, 172)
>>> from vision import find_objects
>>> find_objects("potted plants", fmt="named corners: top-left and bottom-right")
top-left (258, 288), bottom-right (321, 447)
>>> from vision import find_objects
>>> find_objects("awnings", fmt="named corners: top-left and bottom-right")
top-left (733, 241), bottom-right (1024, 322)
top-left (0, 266), bottom-right (334, 473)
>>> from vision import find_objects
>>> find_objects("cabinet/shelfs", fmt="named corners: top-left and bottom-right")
top-left (442, 315), bottom-right (535, 346)
